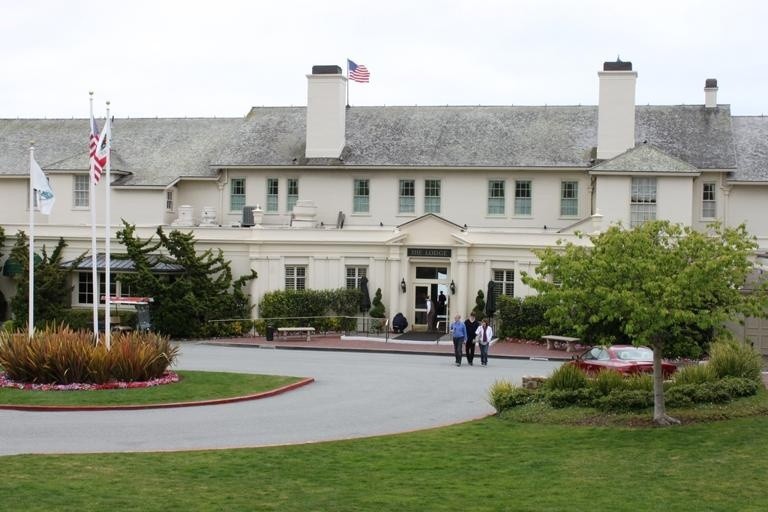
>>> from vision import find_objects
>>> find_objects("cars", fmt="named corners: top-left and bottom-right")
top-left (561, 344), bottom-right (677, 384)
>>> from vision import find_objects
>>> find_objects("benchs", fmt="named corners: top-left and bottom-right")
top-left (540, 334), bottom-right (582, 352)
top-left (110, 316), bottom-right (133, 334)
top-left (275, 326), bottom-right (315, 342)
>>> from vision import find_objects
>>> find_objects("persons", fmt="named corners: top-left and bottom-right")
top-left (424, 295), bottom-right (436, 335)
top-left (464, 312), bottom-right (480, 367)
top-left (474, 320), bottom-right (493, 366)
top-left (448, 314), bottom-right (468, 368)
top-left (439, 290), bottom-right (446, 306)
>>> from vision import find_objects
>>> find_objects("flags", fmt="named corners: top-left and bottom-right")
top-left (93, 117), bottom-right (110, 167)
top-left (89, 113), bottom-right (107, 186)
top-left (348, 61), bottom-right (369, 83)
top-left (31, 158), bottom-right (56, 215)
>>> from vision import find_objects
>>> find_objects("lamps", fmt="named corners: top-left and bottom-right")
top-left (450, 279), bottom-right (456, 295)
top-left (401, 276), bottom-right (408, 294)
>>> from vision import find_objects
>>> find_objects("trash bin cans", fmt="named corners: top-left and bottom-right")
top-left (113, 326), bottom-right (132, 336)
top-left (266, 324), bottom-right (274, 341)
